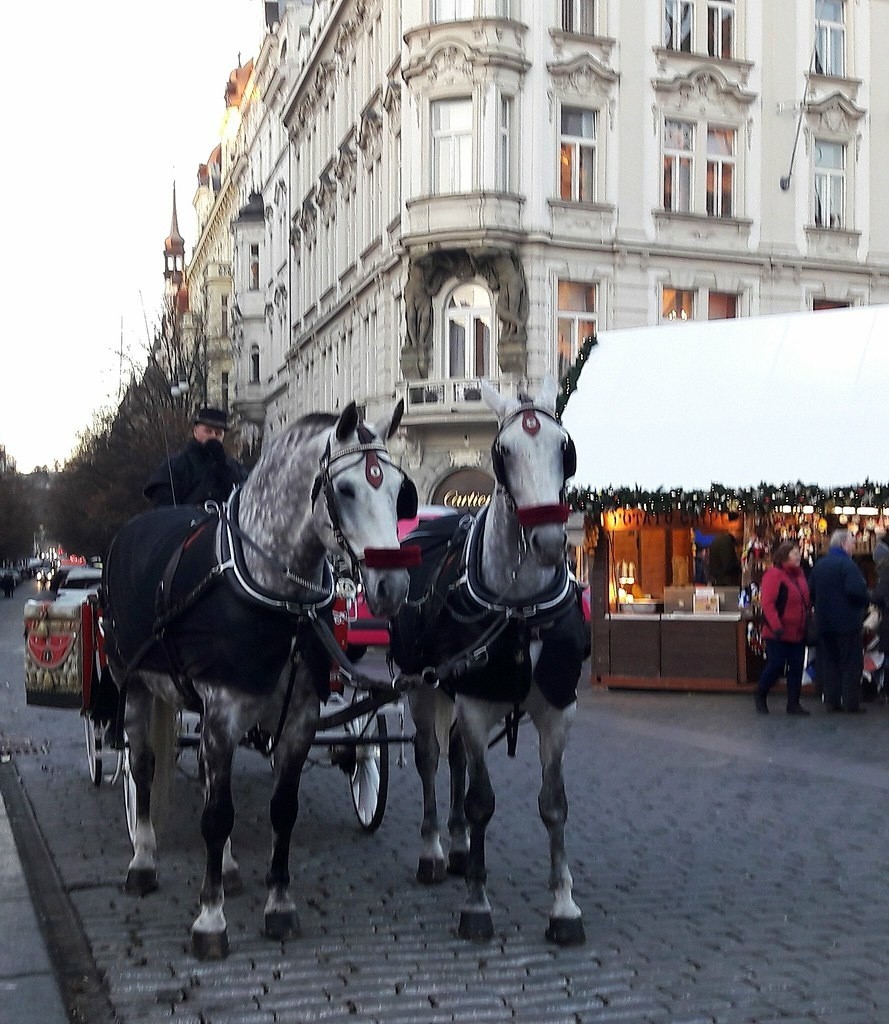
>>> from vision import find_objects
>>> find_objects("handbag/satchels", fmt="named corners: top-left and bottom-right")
top-left (806, 614), bottom-right (823, 647)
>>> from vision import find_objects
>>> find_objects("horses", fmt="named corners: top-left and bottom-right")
top-left (390, 373), bottom-right (592, 947)
top-left (103, 397), bottom-right (410, 960)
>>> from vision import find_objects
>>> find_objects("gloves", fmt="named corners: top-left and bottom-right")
top-left (205, 439), bottom-right (226, 460)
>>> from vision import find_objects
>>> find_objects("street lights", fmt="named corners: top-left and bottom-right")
top-left (171, 379), bottom-right (189, 436)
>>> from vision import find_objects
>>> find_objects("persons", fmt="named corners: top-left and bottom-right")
top-left (806, 528), bottom-right (871, 714)
top-left (142, 406), bottom-right (244, 508)
top-left (695, 532), bottom-right (745, 587)
top-left (0, 565), bottom-right (36, 598)
top-left (754, 541), bottom-right (811, 716)
top-left (859, 527), bottom-right (889, 702)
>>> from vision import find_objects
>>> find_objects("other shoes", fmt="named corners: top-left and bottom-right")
top-left (788, 704), bottom-right (809, 715)
top-left (827, 707), bottom-right (842, 712)
top-left (756, 695), bottom-right (769, 713)
top-left (847, 708), bottom-right (866, 713)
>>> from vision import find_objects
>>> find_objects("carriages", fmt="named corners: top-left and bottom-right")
top-left (78, 380), bottom-right (591, 961)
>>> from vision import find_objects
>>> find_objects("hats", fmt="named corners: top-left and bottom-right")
top-left (873, 542), bottom-right (889, 561)
top-left (195, 408), bottom-right (229, 430)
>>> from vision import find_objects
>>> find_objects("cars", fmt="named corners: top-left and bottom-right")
top-left (57, 569), bottom-right (102, 604)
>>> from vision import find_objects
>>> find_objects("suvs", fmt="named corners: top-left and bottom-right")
top-left (49, 565), bottom-right (83, 590)
